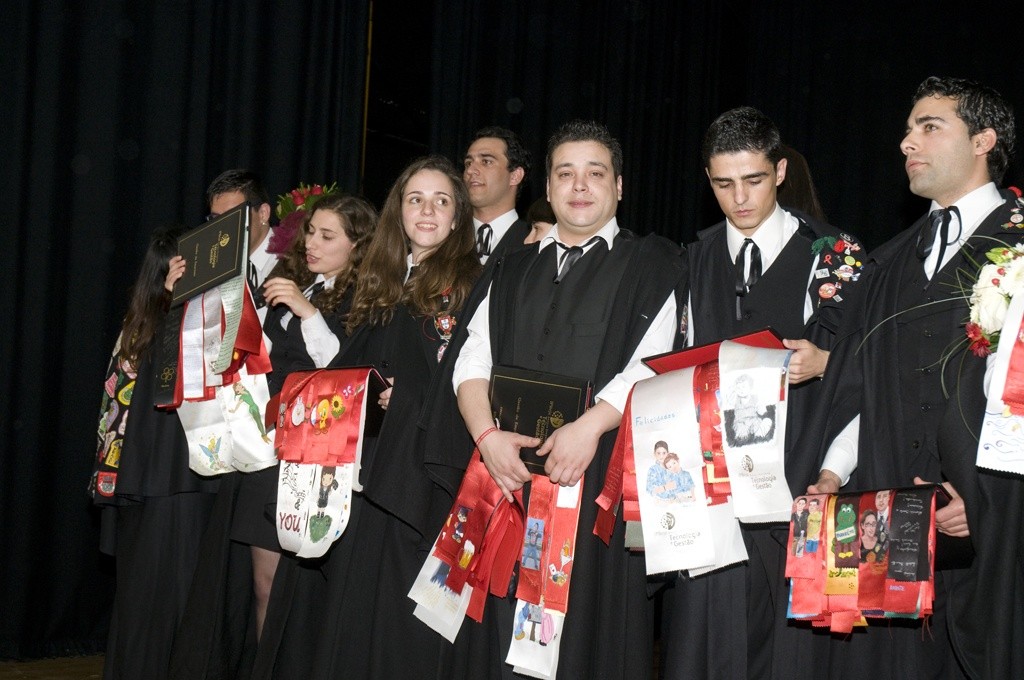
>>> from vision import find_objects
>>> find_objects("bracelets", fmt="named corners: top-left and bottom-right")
top-left (475, 428), bottom-right (499, 445)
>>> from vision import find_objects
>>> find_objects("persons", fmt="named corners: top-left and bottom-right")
top-left (85, 77), bottom-right (1023, 680)
top-left (875, 489), bottom-right (891, 531)
top-left (859, 509), bottom-right (887, 563)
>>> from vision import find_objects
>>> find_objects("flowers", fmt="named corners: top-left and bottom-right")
top-left (276, 182), bottom-right (340, 216)
top-left (857, 234), bottom-right (1024, 443)
